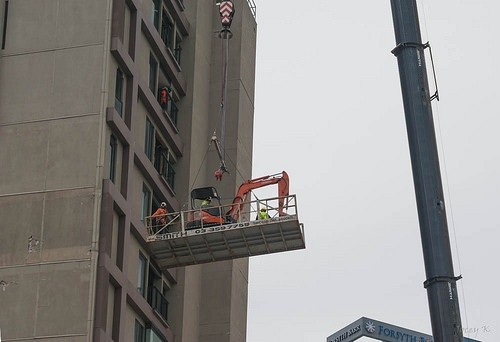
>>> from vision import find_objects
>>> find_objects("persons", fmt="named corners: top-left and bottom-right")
top-left (255, 207), bottom-right (271, 220)
top-left (214, 169), bottom-right (223, 182)
top-left (160, 87), bottom-right (169, 112)
top-left (200, 195), bottom-right (213, 212)
top-left (147, 202), bottom-right (168, 234)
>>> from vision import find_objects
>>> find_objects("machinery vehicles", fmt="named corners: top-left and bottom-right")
top-left (187, 169), bottom-right (291, 231)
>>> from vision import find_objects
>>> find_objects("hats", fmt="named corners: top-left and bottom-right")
top-left (261, 206), bottom-right (266, 210)
top-left (161, 202), bottom-right (166, 206)
